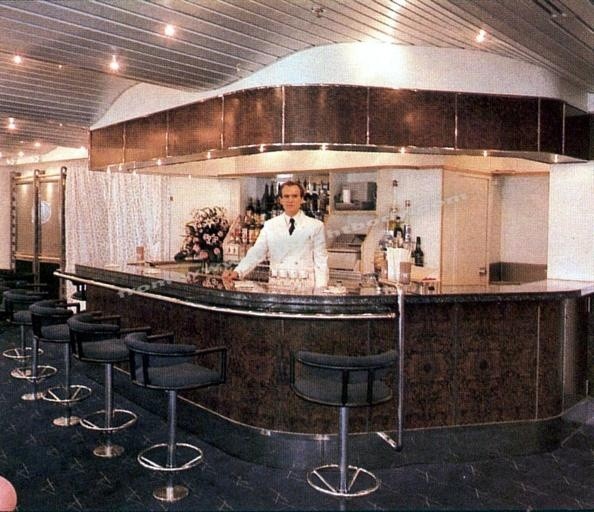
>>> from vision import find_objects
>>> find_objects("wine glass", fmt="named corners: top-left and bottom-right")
top-left (268, 264), bottom-right (309, 291)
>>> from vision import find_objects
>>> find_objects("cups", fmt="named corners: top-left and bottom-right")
top-left (136, 246), bottom-right (144, 261)
top-left (343, 189), bottom-right (351, 203)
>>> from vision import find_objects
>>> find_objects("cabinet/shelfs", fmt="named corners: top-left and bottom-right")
top-left (222, 171), bottom-right (330, 268)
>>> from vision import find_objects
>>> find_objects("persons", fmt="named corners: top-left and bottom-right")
top-left (222, 181), bottom-right (329, 288)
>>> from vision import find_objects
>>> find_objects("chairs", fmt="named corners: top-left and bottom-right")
top-left (66, 312), bottom-right (174, 459)
top-left (120, 330), bottom-right (226, 477)
top-left (1, 279), bottom-right (85, 404)
top-left (289, 348), bottom-right (399, 511)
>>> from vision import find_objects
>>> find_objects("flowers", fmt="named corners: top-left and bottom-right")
top-left (176, 205), bottom-right (230, 261)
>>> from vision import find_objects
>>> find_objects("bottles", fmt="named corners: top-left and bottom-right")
top-left (242, 177), bottom-right (329, 243)
top-left (388, 179), bottom-right (424, 267)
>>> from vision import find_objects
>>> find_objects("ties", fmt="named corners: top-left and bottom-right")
top-left (289, 218), bottom-right (295, 235)
